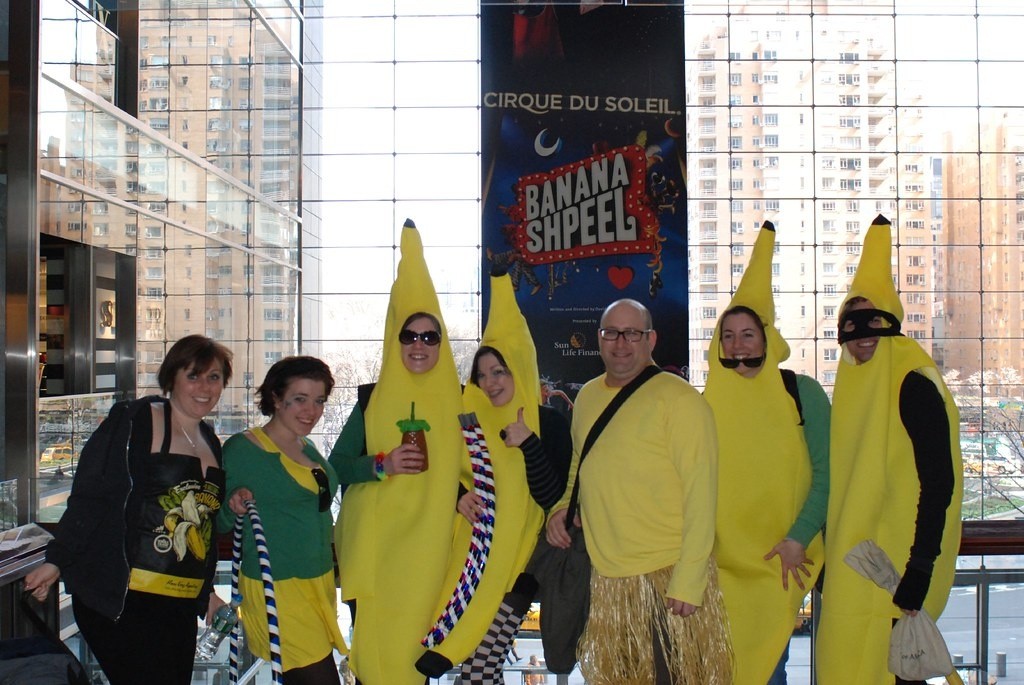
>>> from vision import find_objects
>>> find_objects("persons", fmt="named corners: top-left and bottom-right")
top-left (328, 213), bottom-right (963, 684)
top-left (26, 334), bottom-right (237, 685)
top-left (205, 356), bottom-right (339, 685)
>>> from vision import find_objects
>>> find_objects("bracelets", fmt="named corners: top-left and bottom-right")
top-left (376, 453), bottom-right (385, 475)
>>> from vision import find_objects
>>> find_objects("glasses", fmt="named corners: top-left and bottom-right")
top-left (311, 468), bottom-right (331, 513)
top-left (717, 339), bottom-right (765, 369)
top-left (398, 330), bottom-right (440, 346)
top-left (599, 328), bottom-right (650, 342)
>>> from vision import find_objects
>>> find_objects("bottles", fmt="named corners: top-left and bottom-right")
top-left (397, 419), bottom-right (430, 472)
top-left (195, 594), bottom-right (243, 660)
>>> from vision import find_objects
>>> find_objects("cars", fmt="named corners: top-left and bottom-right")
top-left (519, 605), bottom-right (541, 635)
top-left (964, 455), bottom-right (1014, 474)
top-left (41, 447), bottom-right (78, 460)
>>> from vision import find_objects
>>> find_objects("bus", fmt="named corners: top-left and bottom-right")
top-left (956, 396), bottom-right (1024, 459)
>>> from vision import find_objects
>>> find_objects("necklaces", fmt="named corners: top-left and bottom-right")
top-left (179, 422), bottom-right (197, 448)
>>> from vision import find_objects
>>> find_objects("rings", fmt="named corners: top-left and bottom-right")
top-left (499, 430), bottom-right (507, 440)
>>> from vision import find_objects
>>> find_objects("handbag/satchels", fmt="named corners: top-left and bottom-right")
top-left (523, 524), bottom-right (592, 675)
